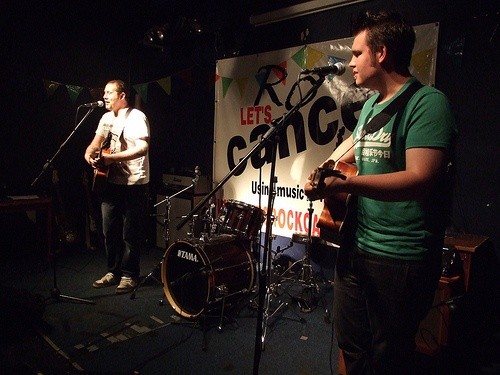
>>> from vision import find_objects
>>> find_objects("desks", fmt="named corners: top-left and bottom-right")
top-left (317, 220), bottom-right (490, 375)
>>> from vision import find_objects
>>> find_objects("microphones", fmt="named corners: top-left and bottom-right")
top-left (312, 61), bottom-right (347, 76)
top-left (84, 100), bottom-right (105, 107)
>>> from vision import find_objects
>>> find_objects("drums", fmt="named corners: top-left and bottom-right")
top-left (160, 233), bottom-right (257, 321)
top-left (218, 198), bottom-right (267, 239)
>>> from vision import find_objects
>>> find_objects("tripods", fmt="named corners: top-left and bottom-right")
top-left (249, 199), bottom-right (332, 352)
top-left (28, 106), bottom-right (97, 306)
top-left (170, 256), bottom-right (235, 352)
top-left (131, 181), bottom-right (196, 303)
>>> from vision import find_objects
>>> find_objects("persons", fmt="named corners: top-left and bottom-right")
top-left (85, 80), bottom-right (151, 291)
top-left (305, 12), bottom-right (455, 375)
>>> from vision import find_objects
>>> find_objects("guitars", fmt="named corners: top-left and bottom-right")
top-left (89, 130), bottom-right (112, 193)
top-left (308, 160), bottom-right (358, 245)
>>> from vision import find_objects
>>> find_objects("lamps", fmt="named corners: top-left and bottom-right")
top-left (249, 0), bottom-right (369, 29)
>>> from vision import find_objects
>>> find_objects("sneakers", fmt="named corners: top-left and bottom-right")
top-left (93, 273), bottom-right (120, 287)
top-left (116, 272), bottom-right (138, 292)
